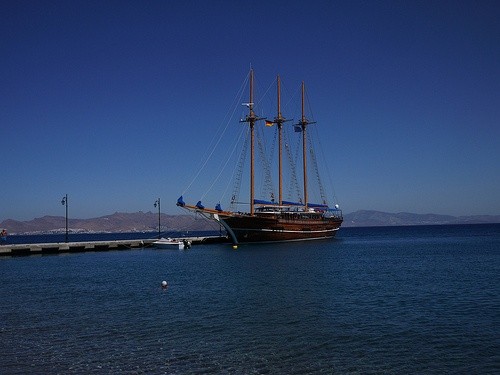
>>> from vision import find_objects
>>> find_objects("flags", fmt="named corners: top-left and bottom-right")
top-left (294, 126), bottom-right (303, 132)
top-left (265, 120), bottom-right (274, 126)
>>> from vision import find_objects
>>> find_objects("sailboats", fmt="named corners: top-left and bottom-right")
top-left (177, 68), bottom-right (344, 245)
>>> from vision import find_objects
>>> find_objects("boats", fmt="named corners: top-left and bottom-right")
top-left (153, 237), bottom-right (193, 251)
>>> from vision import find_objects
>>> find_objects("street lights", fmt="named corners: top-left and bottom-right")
top-left (61, 193), bottom-right (70, 242)
top-left (154, 197), bottom-right (161, 237)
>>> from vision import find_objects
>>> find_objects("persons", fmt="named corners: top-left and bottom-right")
top-left (0, 229), bottom-right (8, 245)
